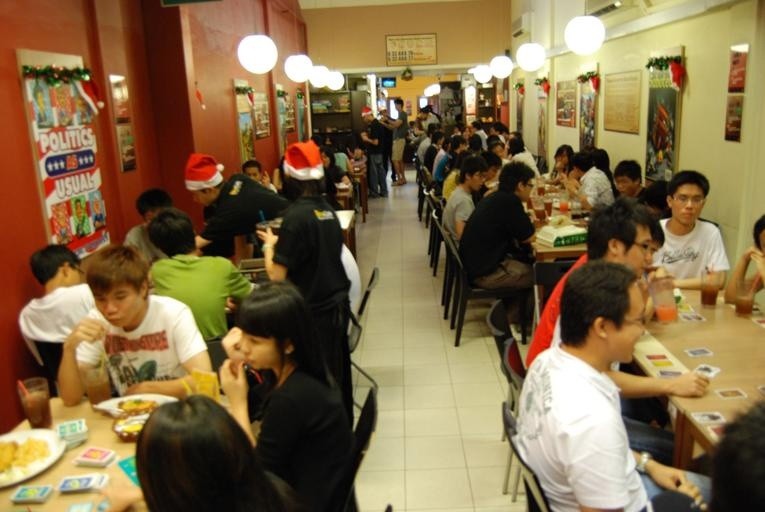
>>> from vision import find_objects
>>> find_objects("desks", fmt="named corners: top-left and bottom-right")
top-left (609, 283), bottom-right (765, 469)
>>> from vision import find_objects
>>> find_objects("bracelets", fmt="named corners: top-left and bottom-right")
top-left (638, 449), bottom-right (652, 473)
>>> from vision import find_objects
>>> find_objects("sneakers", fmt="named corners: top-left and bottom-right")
top-left (403, 180), bottom-right (406, 184)
top-left (391, 175), bottom-right (396, 182)
top-left (368, 194), bottom-right (380, 199)
top-left (391, 181), bottom-right (403, 185)
top-left (379, 193), bottom-right (388, 197)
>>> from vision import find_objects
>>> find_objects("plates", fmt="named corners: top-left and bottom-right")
top-left (0, 429), bottom-right (67, 489)
top-left (92, 394), bottom-right (179, 418)
top-left (553, 201), bottom-right (584, 209)
top-left (545, 192), bottom-right (561, 198)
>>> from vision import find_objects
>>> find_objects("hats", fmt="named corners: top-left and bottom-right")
top-left (184, 153), bottom-right (225, 191)
top-left (362, 106), bottom-right (373, 116)
top-left (284, 142), bottom-right (324, 180)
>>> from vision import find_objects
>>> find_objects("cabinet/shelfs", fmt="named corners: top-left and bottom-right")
top-left (309, 91), bottom-right (368, 149)
top-left (439, 81), bottom-right (463, 128)
top-left (466, 76), bottom-right (498, 124)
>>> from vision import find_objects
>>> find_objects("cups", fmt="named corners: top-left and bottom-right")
top-left (257, 220), bottom-right (274, 232)
top-left (531, 198), bottom-right (546, 218)
top-left (700, 270), bottom-right (719, 310)
top-left (570, 200), bottom-right (582, 222)
top-left (16, 377), bottom-right (55, 429)
top-left (648, 278), bottom-right (678, 325)
top-left (544, 200), bottom-right (553, 218)
top-left (81, 363), bottom-right (112, 411)
top-left (527, 196), bottom-right (533, 210)
top-left (559, 192), bottom-right (569, 212)
top-left (734, 279), bottom-right (755, 316)
top-left (57, 373), bottom-right (82, 407)
top-left (537, 176), bottom-right (545, 197)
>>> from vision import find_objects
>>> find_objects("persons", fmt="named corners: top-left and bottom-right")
top-left (219, 279), bottom-right (357, 511)
top-left (17, 243), bottom-right (106, 397)
top-left (407, 103), bottom-right (663, 339)
top-left (518, 260), bottom-right (713, 511)
top-left (123, 98), bottom-right (396, 355)
top-left (723, 214), bottom-right (765, 303)
top-left (256, 140), bottom-right (353, 433)
top-left (57, 246), bottom-right (220, 407)
top-left (525, 197), bottom-right (710, 464)
top-left (135, 396), bottom-right (307, 512)
top-left (652, 171), bottom-right (729, 292)
top-left (706, 400), bottom-right (764, 511)
top-left (380, 99), bottom-right (408, 186)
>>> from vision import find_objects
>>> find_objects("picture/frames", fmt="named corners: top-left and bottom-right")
top-left (384, 33), bottom-right (438, 66)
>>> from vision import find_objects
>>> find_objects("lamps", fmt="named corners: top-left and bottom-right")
top-left (469, 1), bottom-right (605, 84)
top-left (236, 0), bottom-right (345, 90)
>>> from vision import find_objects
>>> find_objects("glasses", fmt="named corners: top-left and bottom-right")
top-left (474, 174), bottom-right (487, 179)
top-left (527, 183), bottom-right (534, 188)
top-left (634, 242), bottom-right (650, 252)
top-left (671, 195), bottom-right (706, 207)
top-left (624, 316), bottom-right (646, 328)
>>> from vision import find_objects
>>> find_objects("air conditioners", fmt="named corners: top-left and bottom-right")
top-left (512, 12), bottom-right (529, 39)
top-left (586, 0), bottom-right (632, 18)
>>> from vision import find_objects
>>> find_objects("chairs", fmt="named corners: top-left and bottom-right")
top-left (34, 341), bottom-right (64, 396)
top-left (498, 402), bottom-right (664, 512)
top-left (502, 338), bottom-right (605, 500)
top-left (348, 268), bottom-right (381, 420)
top-left (533, 258), bottom-right (584, 331)
top-left (487, 298), bottom-right (546, 441)
top-left (350, 384), bottom-right (378, 512)
top-left (206, 338), bottom-right (230, 373)
top-left (412, 153), bottom-right (527, 347)
